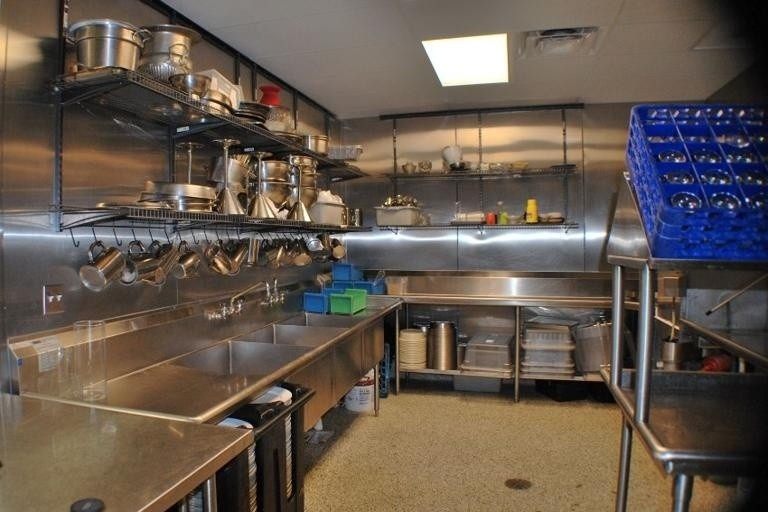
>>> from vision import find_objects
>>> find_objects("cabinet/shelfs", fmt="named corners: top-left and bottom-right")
top-left (53, 0), bottom-right (372, 293)
top-left (378, 103), bottom-right (582, 234)
top-left (601, 174), bottom-right (767, 512)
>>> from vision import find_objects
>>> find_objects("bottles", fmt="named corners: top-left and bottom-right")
top-left (525, 193), bottom-right (538, 224)
top-left (496, 201), bottom-right (505, 223)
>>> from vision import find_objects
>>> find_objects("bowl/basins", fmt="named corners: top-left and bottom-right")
top-left (267, 106), bottom-right (302, 143)
top-left (508, 215), bottom-right (521, 225)
top-left (166, 67), bottom-right (211, 101)
top-left (658, 148), bottom-right (768, 210)
top-left (398, 320), bottom-right (459, 371)
top-left (450, 162), bottom-right (502, 172)
top-left (199, 87), bottom-right (236, 115)
top-left (136, 180), bottom-right (218, 213)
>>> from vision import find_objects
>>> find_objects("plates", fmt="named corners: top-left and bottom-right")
top-left (178, 387), bottom-right (293, 512)
top-left (238, 102), bottom-right (270, 131)
top-left (551, 164), bottom-right (577, 169)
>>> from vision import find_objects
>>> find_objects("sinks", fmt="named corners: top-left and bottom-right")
top-left (279, 312), bottom-right (365, 330)
top-left (228, 324), bottom-right (348, 347)
top-left (172, 341), bottom-right (315, 378)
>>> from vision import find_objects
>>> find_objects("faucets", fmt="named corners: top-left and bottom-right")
top-left (229, 281), bottom-right (270, 311)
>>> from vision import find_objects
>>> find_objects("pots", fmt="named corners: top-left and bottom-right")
top-left (208, 157), bottom-right (248, 188)
top-left (64, 18), bottom-right (153, 71)
top-left (660, 339), bottom-right (693, 364)
top-left (137, 24), bottom-right (202, 81)
top-left (303, 134), bottom-right (329, 156)
top-left (250, 154), bottom-right (317, 214)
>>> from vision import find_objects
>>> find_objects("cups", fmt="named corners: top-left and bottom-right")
top-left (76, 233), bottom-right (348, 290)
top-left (73, 319), bottom-right (107, 401)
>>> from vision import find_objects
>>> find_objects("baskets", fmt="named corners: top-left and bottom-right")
top-left (520, 324), bottom-right (576, 378)
top-left (626, 102), bottom-right (767, 260)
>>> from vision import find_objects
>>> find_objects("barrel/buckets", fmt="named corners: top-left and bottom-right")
top-left (347, 370), bottom-right (377, 414)
top-left (572, 320), bottom-right (612, 373)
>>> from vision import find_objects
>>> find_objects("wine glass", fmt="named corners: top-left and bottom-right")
top-left (208, 138), bottom-right (246, 215)
top-left (286, 162), bottom-right (313, 223)
top-left (449, 200), bottom-right (461, 225)
top-left (245, 152), bottom-right (281, 220)
